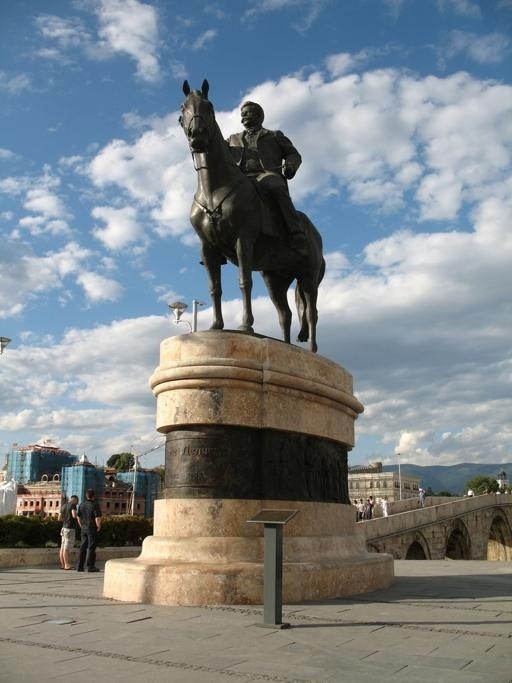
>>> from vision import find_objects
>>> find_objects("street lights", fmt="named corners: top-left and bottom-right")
top-left (168, 299), bottom-right (207, 333)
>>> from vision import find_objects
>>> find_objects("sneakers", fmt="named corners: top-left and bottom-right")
top-left (60, 567), bottom-right (100, 572)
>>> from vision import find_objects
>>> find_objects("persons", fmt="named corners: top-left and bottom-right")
top-left (352, 495), bottom-right (389, 522)
top-left (57, 494), bottom-right (79, 570)
top-left (418, 488), bottom-right (426, 508)
top-left (482, 488), bottom-right (510, 496)
top-left (467, 488), bottom-right (474, 497)
top-left (199, 100), bottom-right (309, 266)
top-left (76, 489), bottom-right (102, 572)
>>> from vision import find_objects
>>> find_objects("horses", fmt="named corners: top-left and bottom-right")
top-left (175, 79), bottom-right (325, 354)
